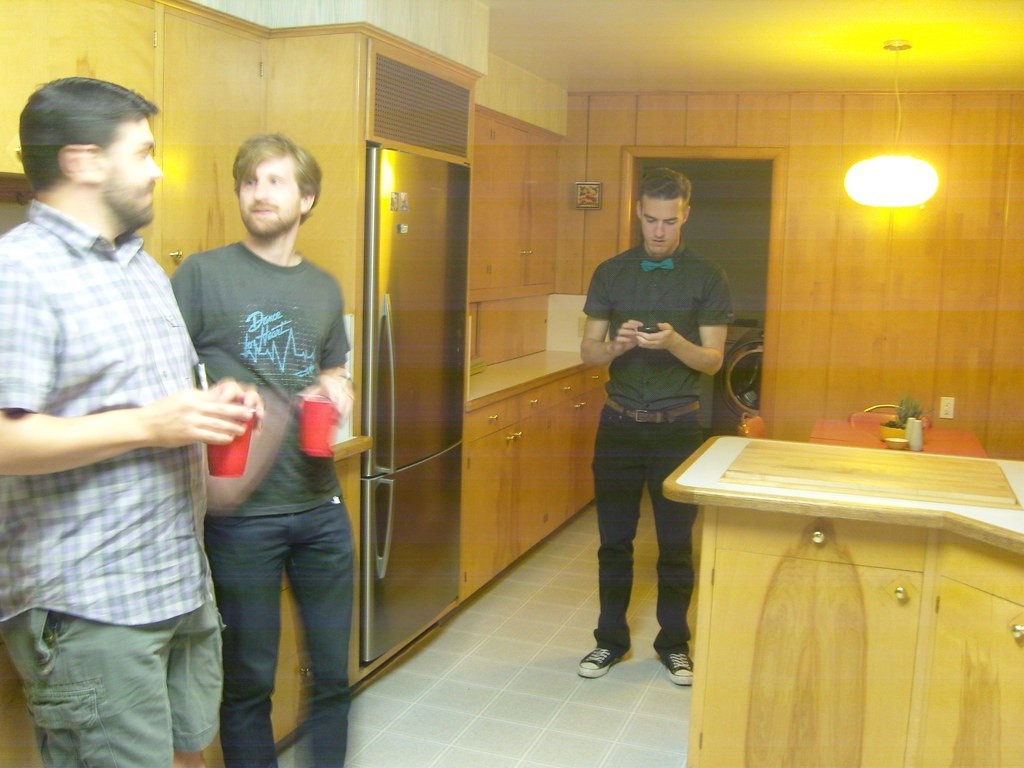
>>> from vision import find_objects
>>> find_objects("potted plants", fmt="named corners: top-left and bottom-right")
top-left (880, 395), bottom-right (934, 440)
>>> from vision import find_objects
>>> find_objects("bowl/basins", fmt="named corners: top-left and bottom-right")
top-left (885, 438), bottom-right (909, 449)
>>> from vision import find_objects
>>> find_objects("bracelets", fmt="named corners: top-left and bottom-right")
top-left (339, 372), bottom-right (353, 383)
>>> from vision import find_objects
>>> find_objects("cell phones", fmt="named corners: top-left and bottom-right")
top-left (637, 326), bottom-right (659, 333)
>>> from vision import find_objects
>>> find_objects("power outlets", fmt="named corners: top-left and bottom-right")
top-left (939, 397), bottom-right (954, 418)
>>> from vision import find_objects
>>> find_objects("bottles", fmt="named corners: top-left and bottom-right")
top-left (907, 417), bottom-right (923, 450)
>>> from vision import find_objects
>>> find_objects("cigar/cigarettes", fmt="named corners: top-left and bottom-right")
top-left (198, 363), bottom-right (208, 391)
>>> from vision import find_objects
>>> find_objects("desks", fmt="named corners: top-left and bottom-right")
top-left (809, 420), bottom-right (988, 460)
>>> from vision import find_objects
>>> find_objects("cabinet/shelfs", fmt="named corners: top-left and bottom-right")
top-left (469, 107), bottom-right (563, 298)
top-left (689, 508), bottom-right (1024, 768)
top-left (1, 0), bottom-right (273, 276)
top-left (463, 348), bottom-right (610, 601)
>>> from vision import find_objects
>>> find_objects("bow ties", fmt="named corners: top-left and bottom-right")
top-left (641, 255), bottom-right (675, 272)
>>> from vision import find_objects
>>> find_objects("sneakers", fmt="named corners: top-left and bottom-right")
top-left (666, 652), bottom-right (693, 684)
top-left (578, 648), bottom-right (621, 677)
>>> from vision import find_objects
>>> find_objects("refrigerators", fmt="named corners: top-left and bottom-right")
top-left (360, 145), bottom-right (472, 661)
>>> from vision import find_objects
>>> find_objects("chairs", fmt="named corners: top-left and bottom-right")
top-left (848, 403), bottom-right (931, 429)
top-left (736, 412), bottom-right (766, 439)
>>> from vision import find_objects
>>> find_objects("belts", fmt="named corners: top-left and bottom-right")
top-left (605, 397), bottom-right (700, 422)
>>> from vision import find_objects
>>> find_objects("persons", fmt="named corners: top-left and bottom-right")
top-left (171, 135), bottom-right (353, 768)
top-left (578, 167), bottom-right (733, 685)
top-left (0, 78), bottom-right (267, 768)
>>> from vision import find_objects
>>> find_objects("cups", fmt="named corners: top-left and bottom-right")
top-left (300, 394), bottom-right (339, 459)
top-left (207, 409), bottom-right (256, 478)
